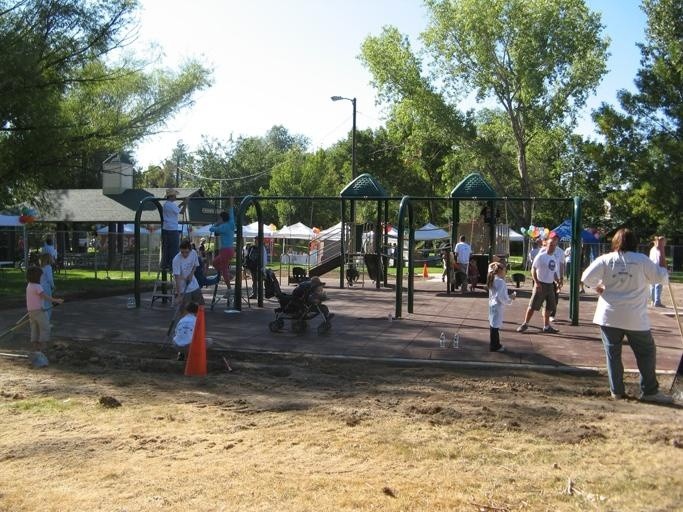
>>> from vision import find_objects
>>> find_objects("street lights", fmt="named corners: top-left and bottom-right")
top-left (330, 93), bottom-right (358, 226)
top-left (19, 205), bottom-right (37, 270)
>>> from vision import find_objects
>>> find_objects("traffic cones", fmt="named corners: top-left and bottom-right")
top-left (422, 264), bottom-right (428, 278)
top-left (176, 303), bottom-right (211, 376)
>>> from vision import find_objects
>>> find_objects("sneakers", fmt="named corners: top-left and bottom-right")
top-left (542, 325), bottom-right (559, 334)
top-left (517, 322), bottom-right (528, 332)
top-left (176, 352), bottom-right (185, 361)
top-left (638, 390), bottom-right (674, 404)
top-left (608, 385), bottom-right (622, 400)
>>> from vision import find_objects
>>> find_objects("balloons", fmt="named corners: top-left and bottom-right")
top-left (313, 227), bottom-right (320, 234)
top-left (18, 206), bottom-right (36, 224)
top-left (521, 225), bottom-right (556, 241)
top-left (387, 226), bottom-right (391, 232)
top-left (269, 223), bottom-right (276, 232)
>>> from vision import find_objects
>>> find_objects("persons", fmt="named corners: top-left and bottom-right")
top-left (486, 261), bottom-right (516, 353)
top-left (516, 235), bottom-right (571, 333)
top-left (310, 277), bottom-right (335, 320)
top-left (441, 235), bottom-right (481, 292)
top-left (393, 242), bottom-right (398, 267)
top-left (161, 188), bottom-right (268, 311)
top-left (43, 239), bottom-right (56, 257)
top-left (579, 226), bottom-right (675, 403)
top-left (25, 266), bottom-right (65, 347)
top-left (172, 301), bottom-right (213, 360)
top-left (649, 234), bottom-right (667, 308)
top-left (41, 252), bottom-right (57, 328)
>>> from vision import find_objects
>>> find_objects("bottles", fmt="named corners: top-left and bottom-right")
top-left (387, 312), bottom-right (392, 323)
top-left (439, 332), bottom-right (445, 348)
top-left (452, 333), bottom-right (458, 348)
top-left (126, 297), bottom-right (136, 308)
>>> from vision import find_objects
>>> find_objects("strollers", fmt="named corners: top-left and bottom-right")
top-left (264, 265), bottom-right (334, 334)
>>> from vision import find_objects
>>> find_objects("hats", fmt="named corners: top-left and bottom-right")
top-left (311, 277), bottom-right (326, 289)
top-left (549, 232), bottom-right (559, 238)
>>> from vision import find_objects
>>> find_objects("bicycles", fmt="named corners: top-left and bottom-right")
top-left (16, 253), bottom-right (64, 275)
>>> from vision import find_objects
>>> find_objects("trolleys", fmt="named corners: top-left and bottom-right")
top-left (262, 267), bottom-right (281, 299)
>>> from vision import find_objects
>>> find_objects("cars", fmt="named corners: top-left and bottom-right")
top-left (88, 237), bottom-right (95, 248)
